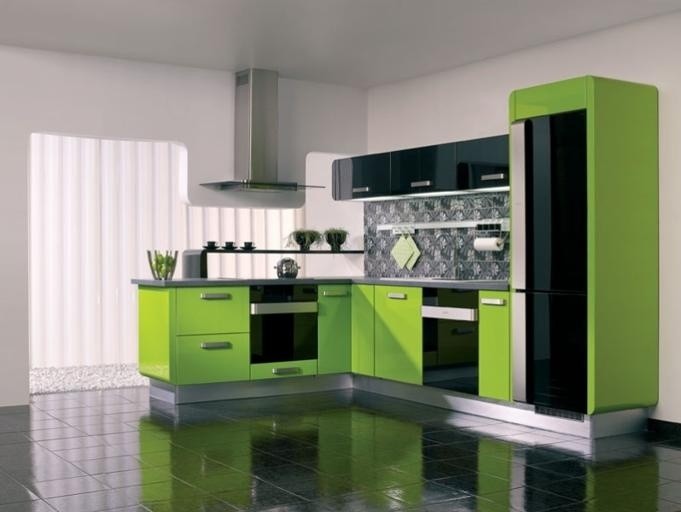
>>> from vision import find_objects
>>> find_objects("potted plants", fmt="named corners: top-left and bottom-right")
top-left (285, 230), bottom-right (323, 251)
top-left (322, 228), bottom-right (349, 252)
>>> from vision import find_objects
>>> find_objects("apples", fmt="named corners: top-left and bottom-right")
top-left (152, 254), bottom-right (173, 277)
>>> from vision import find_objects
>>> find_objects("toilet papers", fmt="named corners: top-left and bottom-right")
top-left (472, 236), bottom-right (503, 254)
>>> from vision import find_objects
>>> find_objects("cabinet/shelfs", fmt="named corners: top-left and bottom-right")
top-left (137, 407), bottom-right (510, 512)
top-left (331, 133), bottom-right (510, 202)
top-left (136, 286), bottom-right (513, 403)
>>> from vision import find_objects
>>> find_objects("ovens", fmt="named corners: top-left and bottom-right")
top-left (249, 285), bottom-right (319, 364)
top-left (422, 288), bottom-right (477, 396)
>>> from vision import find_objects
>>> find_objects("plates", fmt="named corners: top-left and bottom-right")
top-left (203, 246), bottom-right (219, 250)
top-left (241, 246), bottom-right (255, 250)
top-left (221, 246), bottom-right (237, 250)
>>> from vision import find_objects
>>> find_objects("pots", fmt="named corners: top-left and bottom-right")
top-left (277, 258), bottom-right (298, 278)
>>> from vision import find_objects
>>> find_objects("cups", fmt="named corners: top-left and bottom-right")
top-left (207, 241), bottom-right (218, 246)
top-left (244, 242), bottom-right (254, 247)
top-left (225, 241), bottom-right (236, 247)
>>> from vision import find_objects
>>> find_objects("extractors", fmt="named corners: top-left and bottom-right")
top-left (198, 68), bottom-right (326, 193)
